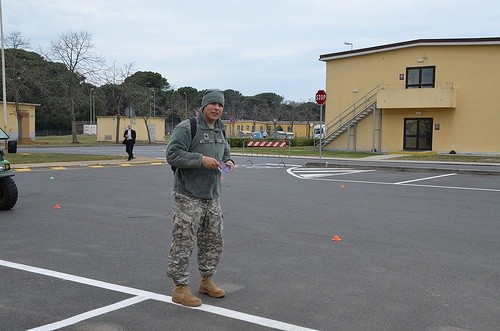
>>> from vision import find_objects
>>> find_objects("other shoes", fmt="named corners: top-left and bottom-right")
top-left (127, 159), bottom-right (131, 161)
top-left (131, 155), bottom-right (133, 159)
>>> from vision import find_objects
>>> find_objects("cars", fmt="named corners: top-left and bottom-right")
top-left (0, 127), bottom-right (19, 210)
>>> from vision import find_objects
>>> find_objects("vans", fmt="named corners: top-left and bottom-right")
top-left (238, 130), bottom-right (294, 139)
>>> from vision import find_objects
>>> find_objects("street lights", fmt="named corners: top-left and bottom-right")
top-left (344, 42), bottom-right (353, 50)
top-left (90, 88), bottom-right (97, 125)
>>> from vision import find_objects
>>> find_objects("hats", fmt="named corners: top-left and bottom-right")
top-left (202, 89), bottom-right (224, 113)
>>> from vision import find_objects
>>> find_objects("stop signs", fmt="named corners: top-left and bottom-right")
top-left (315, 90), bottom-right (327, 105)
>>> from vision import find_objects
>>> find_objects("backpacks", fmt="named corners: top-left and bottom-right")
top-left (171, 117), bottom-right (197, 174)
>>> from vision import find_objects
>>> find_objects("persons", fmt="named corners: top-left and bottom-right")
top-left (122, 124), bottom-right (136, 161)
top-left (166, 89), bottom-right (235, 307)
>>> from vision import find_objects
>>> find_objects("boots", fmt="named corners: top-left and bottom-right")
top-left (172, 285), bottom-right (202, 307)
top-left (199, 275), bottom-right (225, 298)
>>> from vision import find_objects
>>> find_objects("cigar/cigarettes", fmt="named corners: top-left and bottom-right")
top-left (216, 114), bottom-right (223, 122)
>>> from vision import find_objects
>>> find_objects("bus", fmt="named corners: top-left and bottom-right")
top-left (313, 124), bottom-right (326, 140)
